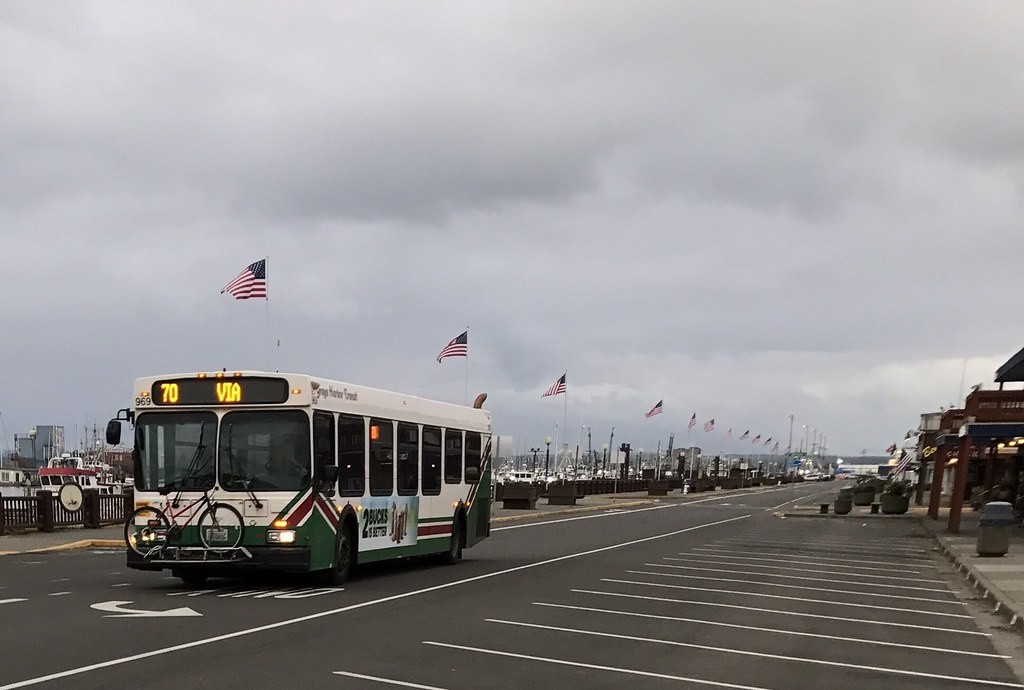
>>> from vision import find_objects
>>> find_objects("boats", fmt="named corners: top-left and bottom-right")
top-left (34, 423), bottom-right (127, 509)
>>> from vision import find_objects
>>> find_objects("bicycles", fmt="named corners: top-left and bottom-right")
top-left (124, 477), bottom-right (245, 562)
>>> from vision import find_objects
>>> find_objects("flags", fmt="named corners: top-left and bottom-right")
top-left (895, 448), bottom-right (912, 476)
top-left (437, 331), bottom-right (468, 363)
top-left (543, 374), bottom-right (565, 397)
top-left (786, 446), bottom-right (790, 450)
top-left (773, 442), bottom-right (778, 449)
top-left (741, 430), bottom-right (750, 439)
top-left (688, 413), bottom-right (696, 428)
top-left (645, 401), bottom-right (662, 417)
top-left (221, 259), bottom-right (266, 300)
top-left (764, 438), bottom-right (771, 445)
top-left (752, 434), bottom-right (760, 442)
top-left (704, 420), bottom-right (714, 431)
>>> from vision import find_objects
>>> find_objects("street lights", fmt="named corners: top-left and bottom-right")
top-left (801, 424), bottom-right (827, 471)
top-left (602, 444), bottom-right (609, 479)
top-left (542, 436), bottom-right (552, 483)
top-left (784, 414), bottom-right (793, 478)
top-left (679, 452), bottom-right (685, 478)
top-left (638, 448), bottom-right (645, 480)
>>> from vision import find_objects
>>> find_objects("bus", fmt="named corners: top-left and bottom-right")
top-left (105, 368), bottom-right (492, 588)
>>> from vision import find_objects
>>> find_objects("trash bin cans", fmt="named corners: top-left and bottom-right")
top-left (977, 501), bottom-right (1015, 557)
